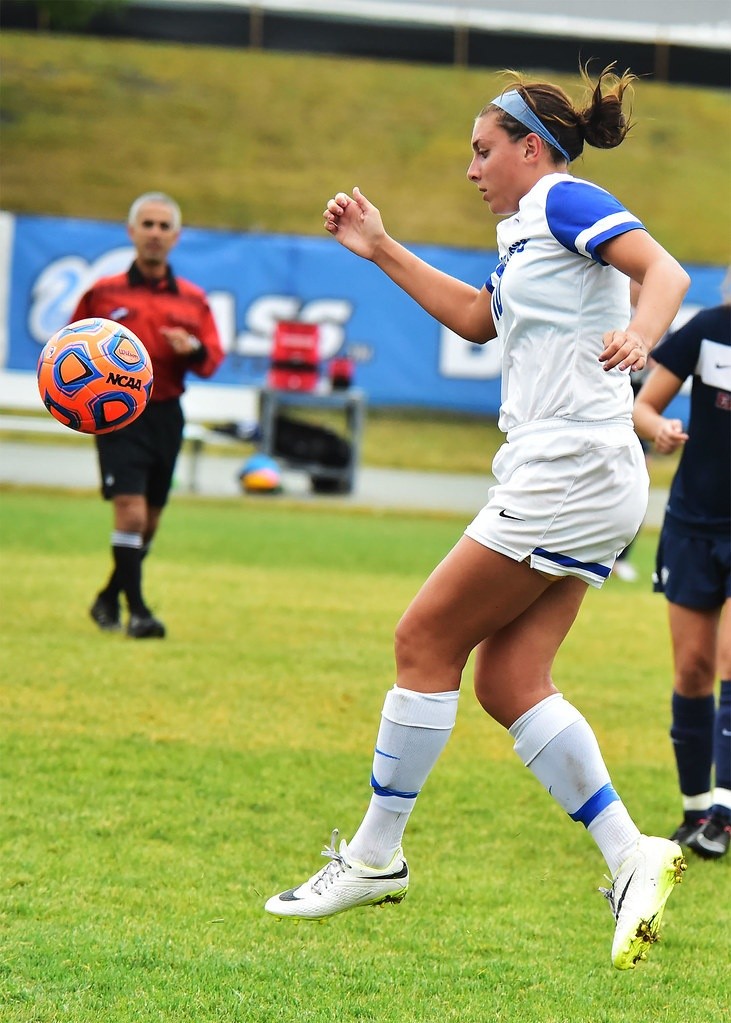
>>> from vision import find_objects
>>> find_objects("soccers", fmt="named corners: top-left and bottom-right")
top-left (36, 316), bottom-right (155, 435)
top-left (234, 453), bottom-right (284, 495)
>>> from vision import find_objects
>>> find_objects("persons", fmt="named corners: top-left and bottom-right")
top-left (66, 188), bottom-right (226, 641)
top-left (261, 56), bottom-right (687, 982)
top-left (626, 280), bottom-right (666, 458)
top-left (631, 262), bottom-right (731, 860)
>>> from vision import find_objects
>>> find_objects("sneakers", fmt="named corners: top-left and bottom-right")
top-left (610, 836), bottom-right (685, 971)
top-left (91, 593), bottom-right (119, 630)
top-left (124, 612), bottom-right (164, 641)
top-left (262, 836), bottom-right (407, 916)
top-left (686, 813), bottom-right (729, 858)
top-left (670, 814), bottom-right (701, 849)
